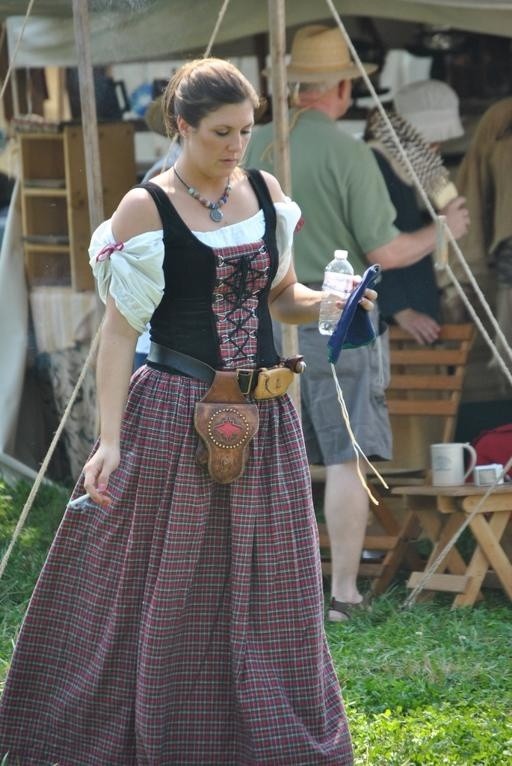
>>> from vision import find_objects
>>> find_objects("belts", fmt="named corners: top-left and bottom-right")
top-left (147, 342), bottom-right (257, 398)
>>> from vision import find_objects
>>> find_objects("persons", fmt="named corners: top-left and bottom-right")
top-left (0, 52), bottom-right (378, 765)
top-left (239, 24), bottom-right (472, 627)
top-left (362, 77), bottom-right (466, 348)
top-left (432, 95), bottom-right (512, 372)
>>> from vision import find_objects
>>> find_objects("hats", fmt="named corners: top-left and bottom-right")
top-left (394, 80), bottom-right (466, 142)
top-left (260, 25), bottom-right (378, 83)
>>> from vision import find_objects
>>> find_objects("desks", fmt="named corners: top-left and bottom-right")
top-left (390, 483), bottom-right (512, 612)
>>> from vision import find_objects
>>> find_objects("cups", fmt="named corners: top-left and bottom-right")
top-left (429, 442), bottom-right (477, 486)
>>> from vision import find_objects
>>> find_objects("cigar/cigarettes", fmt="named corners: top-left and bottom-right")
top-left (65, 487), bottom-right (99, 509)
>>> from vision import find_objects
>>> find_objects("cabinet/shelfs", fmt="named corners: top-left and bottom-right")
top-left (20, 125), bottom-right (137, 294)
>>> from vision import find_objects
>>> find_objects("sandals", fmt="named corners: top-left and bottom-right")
top-left (327, 595), bottom-right (381, 626)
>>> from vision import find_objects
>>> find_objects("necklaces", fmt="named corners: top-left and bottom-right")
top-left (171, 164), bottom-right (232, 222)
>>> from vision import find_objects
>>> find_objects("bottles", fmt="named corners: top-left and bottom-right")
top-left (318, 249), bottom-right (355, 336)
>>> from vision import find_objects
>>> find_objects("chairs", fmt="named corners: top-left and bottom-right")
top-left (309, 324), bottom-right (473, 597)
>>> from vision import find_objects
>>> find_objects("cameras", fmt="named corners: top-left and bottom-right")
top-left (474, 464), bottom-right (505, 487)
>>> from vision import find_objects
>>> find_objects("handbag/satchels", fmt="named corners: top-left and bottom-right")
top-left (466, 424), bottom-right (512, 484)
